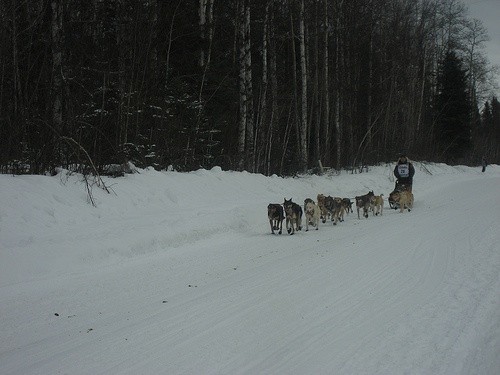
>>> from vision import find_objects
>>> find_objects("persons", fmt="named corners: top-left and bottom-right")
top-left (394, 155), bottom-right (415, 193)
top-left (482, 154), bottom-right (490, 172)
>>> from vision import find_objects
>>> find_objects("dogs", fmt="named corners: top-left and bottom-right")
top-left (317, 193), bottom-right (354, 226)
top-left (304, 198), bottom-right (320, 232)
top-left (268, 203), bottom-right (285, 235)
top-left (283, 198), bottom-right (303, 235)
top-left (390, 190), bottom-right (414, 213)
top-left (355, 190), bottom-right (384, 219)
